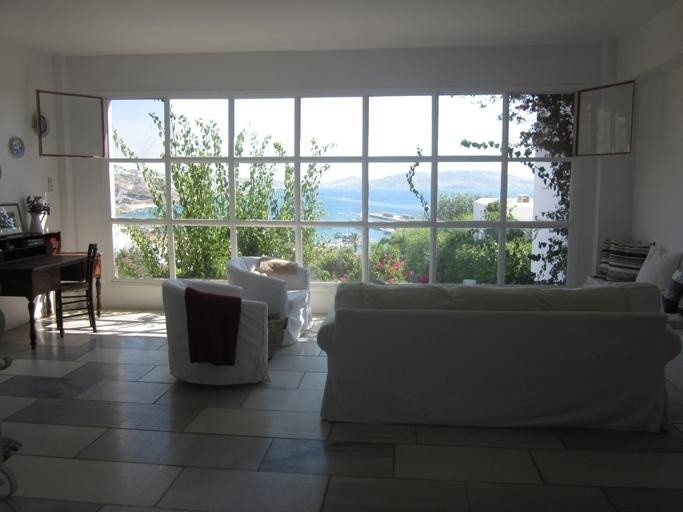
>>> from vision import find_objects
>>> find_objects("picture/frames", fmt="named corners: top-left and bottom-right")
top-left (0, 203), bottom-right (24, 236)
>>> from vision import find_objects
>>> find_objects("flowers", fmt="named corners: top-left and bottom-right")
top-left (27, 194), bottom-right (48, 211)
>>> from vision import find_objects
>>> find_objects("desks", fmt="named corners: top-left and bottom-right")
top-left (0, 233), bottom-right (103, 350)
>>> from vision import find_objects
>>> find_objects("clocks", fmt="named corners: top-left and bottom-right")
top-left (8, 136), bottom-right (25, 158)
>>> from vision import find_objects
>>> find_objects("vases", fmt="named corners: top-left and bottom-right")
top-left (29, 211), bottom-right (44, 233)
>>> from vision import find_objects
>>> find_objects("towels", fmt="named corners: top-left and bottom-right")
top-left (259, 257), bottom-right (298, 273)
top-left (184, 288), bottom-right (240, 365)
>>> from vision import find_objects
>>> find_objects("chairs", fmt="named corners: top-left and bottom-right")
top-left (56, 243), bottom-right (98, 337)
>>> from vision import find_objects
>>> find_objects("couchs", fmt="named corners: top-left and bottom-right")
top-left (225, 254), bottom-right (310, 347)
top-left (162, 278), bottom-right (271, 386)
top-left (316, 281), bottom-right (681, 435)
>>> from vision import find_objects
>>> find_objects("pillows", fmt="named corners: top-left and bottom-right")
top-left (635, 246), bottom-right (682, 289)
top-left (596, 238), bottom-right (641, 278)
top-left (606, 241), bottom-right (654, 283)
